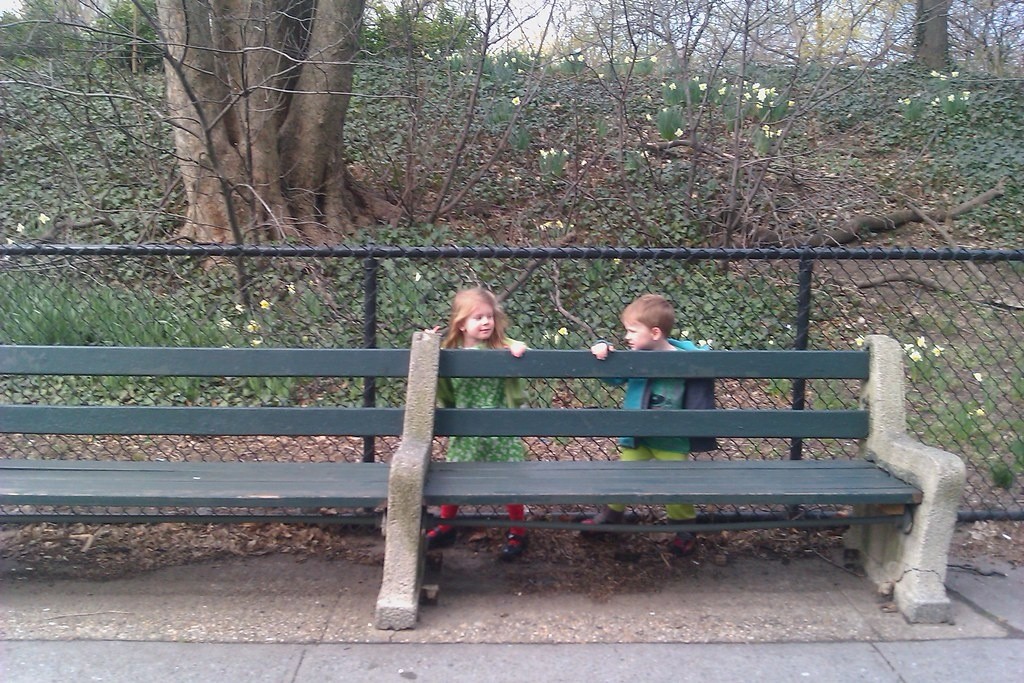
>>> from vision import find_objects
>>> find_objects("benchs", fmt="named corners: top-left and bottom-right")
top-left (0, 332), bottom-right (968, 629)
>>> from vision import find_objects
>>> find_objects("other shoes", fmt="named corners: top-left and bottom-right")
top-left (667, 526), bottom-right (697, 557)
top-left (424, 524), bottom-right (456, 545)
top-left (580, 505), bottom-right (623, 540)
top-left (503, 535), bottom-right (529, 560)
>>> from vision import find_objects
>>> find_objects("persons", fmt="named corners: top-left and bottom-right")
top-left (580, 294), bottom-right (715, 556)
top-left (422, 284), bottom-right (533, 563)
